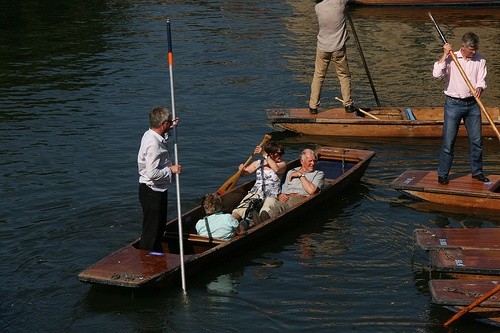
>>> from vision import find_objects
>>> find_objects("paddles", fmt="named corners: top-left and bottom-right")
top-left (444, 283), bottom-right (500, 326)
top-left (218, 133), bottom-right (272, 195)
top-left (334, 95), bottom-right (381, 120)
top-left (428, 11), bottom-right (500, 140)
top-left (167, 18), bottom-right (186, 302)
top-left (345, 6), bottom-right (381, 106)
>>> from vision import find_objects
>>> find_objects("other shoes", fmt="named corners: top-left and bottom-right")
top-left (310, 108), bottom-right (317, 113)
top-left (345, 105), bottom-right (354, 113)
top-left (260, 210), bottom-right (271, 222)
top-left (253, 210), bottom-right (261, 225)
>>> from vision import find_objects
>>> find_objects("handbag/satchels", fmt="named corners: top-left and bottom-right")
top-left (244, 198), bottom-right (264, 220)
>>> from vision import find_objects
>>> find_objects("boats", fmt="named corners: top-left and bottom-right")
top-left (266, 102), bottom-right (500, 137)
top-left (78, 146), bottom-right (376, 295)
top-left (411, 227), bottom-right (500, 326)
top-left (391, 169), bottom-right (500, 210)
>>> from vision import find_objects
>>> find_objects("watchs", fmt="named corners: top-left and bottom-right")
top-left (264, 154), bottom-right (270, 159)
top-left (299, 173), bottom-right (306, 179)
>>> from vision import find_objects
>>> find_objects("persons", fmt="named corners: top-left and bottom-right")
top-left (433, 31), bottom-right (490, 185)
top-left (252, 148), bottom-right (325, 228)
top-left (194, 193), bottom-right (264, 240)
top-left (307, 0), bottom-right (355, 114)
top-left (230, 140), bottom-right (287, 222)
top-left (136, 106), bottom-right (181, 251)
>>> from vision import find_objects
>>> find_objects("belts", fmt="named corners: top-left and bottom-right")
top-left (447, 95), bottom-right (474, 102)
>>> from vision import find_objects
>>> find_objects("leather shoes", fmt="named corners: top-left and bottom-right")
top-left (438, 176), bottom-right (449, 185)
top-left (472, 174), bottom-right (489, 182)
